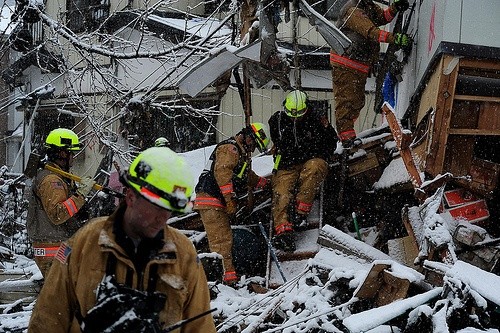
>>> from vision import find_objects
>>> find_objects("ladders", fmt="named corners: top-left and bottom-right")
top-left (265, 183), bottom-right (326, 288)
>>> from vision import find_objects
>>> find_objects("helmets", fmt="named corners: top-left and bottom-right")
top-left (118, 147), bottom-right (194, 213)
top-left (247, 122), bottom-right (271, 153)
top-left (45, 128), bottom-right (80, 151)
top-left (155, 137), bottom-right (170, 148)
top-left (281, 89), bottom-right (309, 117)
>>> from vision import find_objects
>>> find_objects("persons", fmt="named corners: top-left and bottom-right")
top-left (29, 145), bottom-right (215, 333)
top-left (331, 0), bottom-right (414, 147)
top-left (26, 128), bottom-right (96, 278)
top-left (268, 91), bottom-right (339, 253)
top-left (155, 137), bottom-right (169, 147)
top-left (194, 122), bottom-right (272, 289)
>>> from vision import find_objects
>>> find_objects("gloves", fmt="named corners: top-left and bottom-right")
top-left (390, 0), bottom-right (409, 12)
top-left (224, 200), bottom-right (237, 220)
top-left (393, 33), bottom-right (414, 56)
top-left (76, 176), bottom-right (97, 198)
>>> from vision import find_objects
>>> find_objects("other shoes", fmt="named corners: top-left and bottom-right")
top-left (293, 213), bottom-right (308, 232)
top-left (274, 231), bottom-right (296, 252)
top-left (342, 138), bottom-right (362, 148)
top-left (223, 280), bottom-right (239, 290)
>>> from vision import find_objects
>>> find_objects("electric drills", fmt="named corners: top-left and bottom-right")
top-left (381, 28), bottom-right (414, 108)
